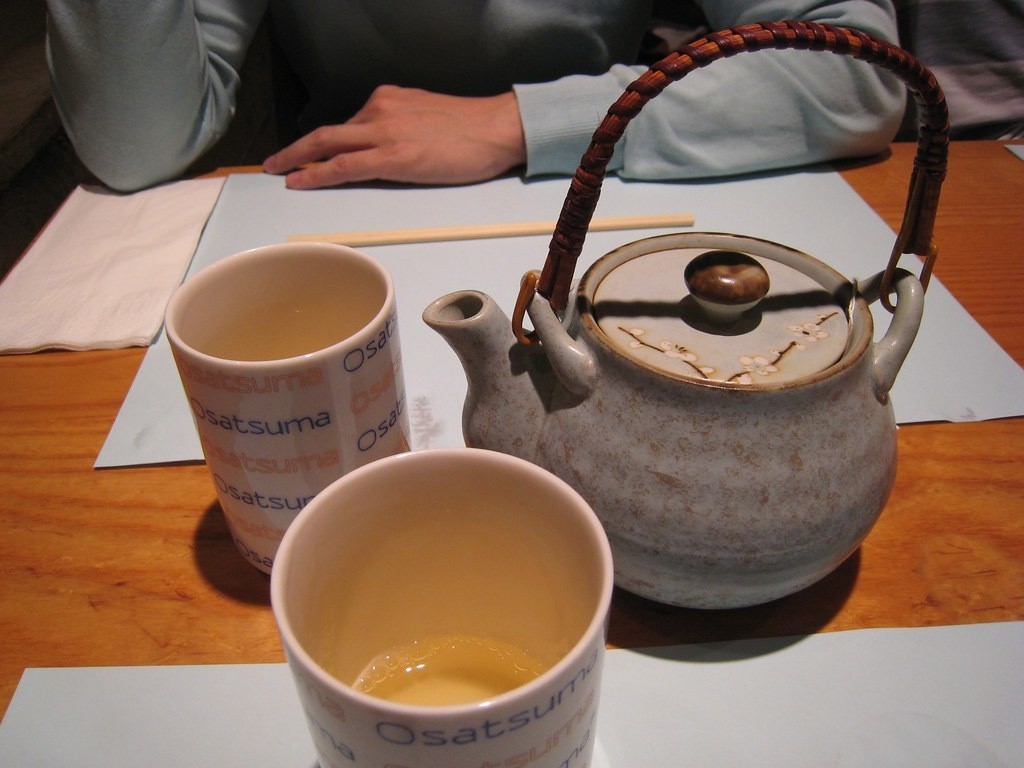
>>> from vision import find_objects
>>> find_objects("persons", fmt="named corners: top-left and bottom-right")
top-left (41, 0), bottom-right (906, 194)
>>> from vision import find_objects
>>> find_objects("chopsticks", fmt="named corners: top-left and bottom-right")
top-left (291, 213), bottom-right (696, 248)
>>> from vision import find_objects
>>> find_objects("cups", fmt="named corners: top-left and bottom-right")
top-left (270, 448), bottom-right (616, 768)
top-left (162, 240), bottom-right (411, 577)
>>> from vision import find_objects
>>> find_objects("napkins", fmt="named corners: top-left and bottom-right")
top-left (0, 176), bottom-right (225, 356)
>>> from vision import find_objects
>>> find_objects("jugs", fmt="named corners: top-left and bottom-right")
top-left (420, 19), bottom-right (950, 611)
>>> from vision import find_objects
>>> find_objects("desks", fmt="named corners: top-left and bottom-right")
top-left (0, 138), bottom-right (1024, 768)
